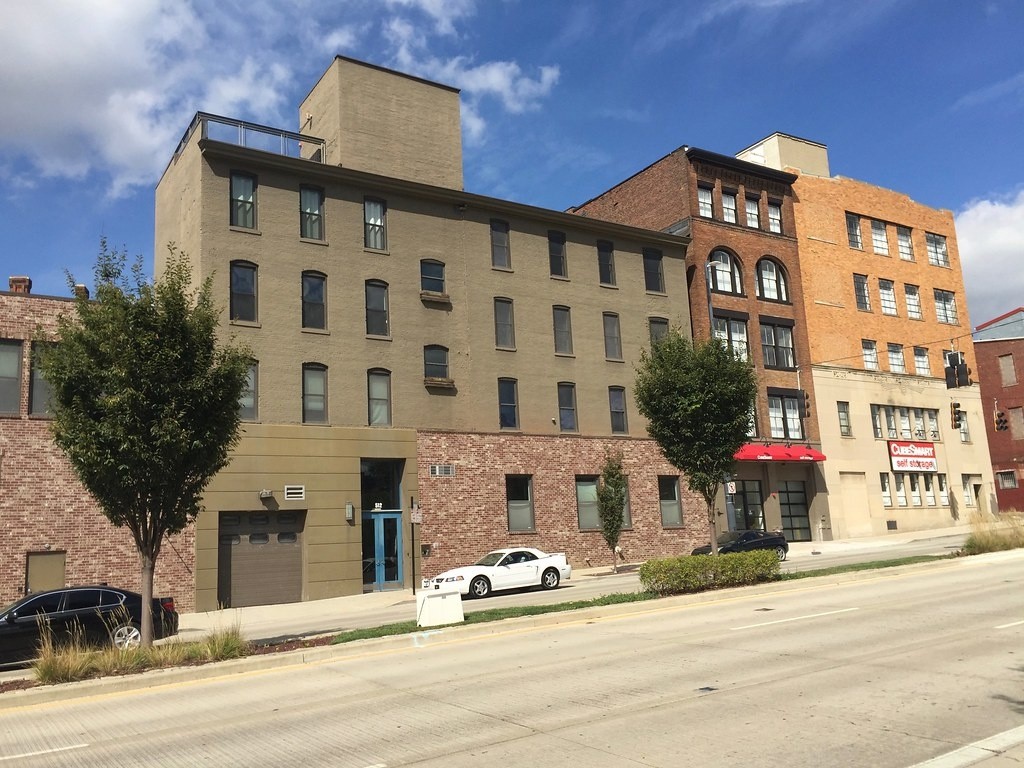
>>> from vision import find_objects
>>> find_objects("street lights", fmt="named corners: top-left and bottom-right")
top-left (705, 260), bottom-right (739, 533)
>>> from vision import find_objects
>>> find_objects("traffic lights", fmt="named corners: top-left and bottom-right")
top-left (950, 401), bottom-right (962, 429)
top-left (994, 410), bottom-right (1008, 432)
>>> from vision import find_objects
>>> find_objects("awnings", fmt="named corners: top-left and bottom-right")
top-left (733, 445), bottom-right (826, 462)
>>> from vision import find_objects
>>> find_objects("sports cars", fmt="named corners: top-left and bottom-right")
top-left (421, 547), bottom-right (572, 601)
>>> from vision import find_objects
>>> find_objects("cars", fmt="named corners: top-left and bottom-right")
top-left (0, 585), bottom-right (179, 667)
top-left (690, 529), bottom-right (789, 562)
